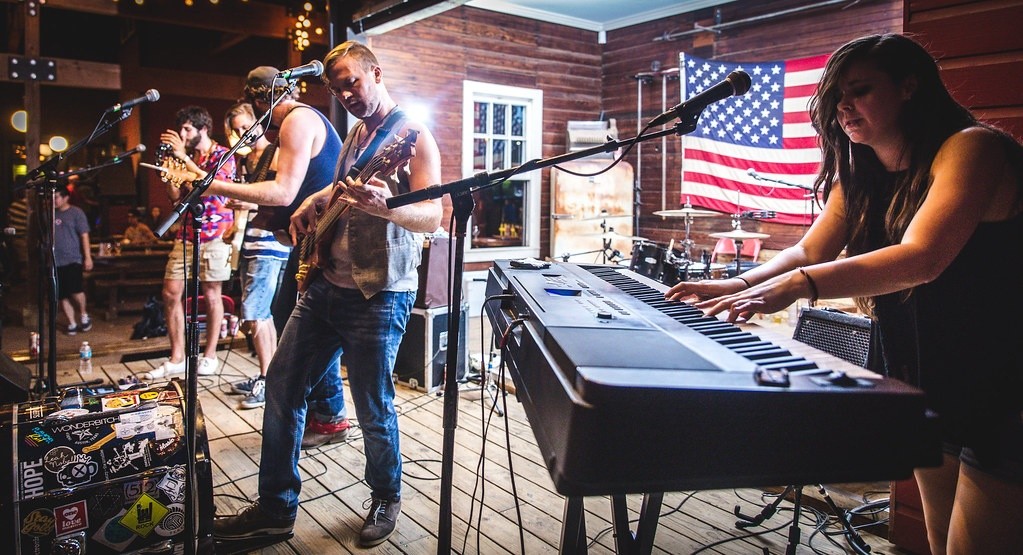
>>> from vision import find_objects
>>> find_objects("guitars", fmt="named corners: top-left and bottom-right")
top-left (224, 80), bottom-right (308, 272)
top-left (270, 127), bottom-right (422, 347)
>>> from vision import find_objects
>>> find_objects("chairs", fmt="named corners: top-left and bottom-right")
top-left (711, 237), bottom-right (761, 263)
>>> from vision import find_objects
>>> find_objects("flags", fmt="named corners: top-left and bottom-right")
top-left (684, 55), bottom-right (837, 226)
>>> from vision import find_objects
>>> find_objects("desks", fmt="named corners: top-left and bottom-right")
top-left (85, 251), bottom-right (171, 279)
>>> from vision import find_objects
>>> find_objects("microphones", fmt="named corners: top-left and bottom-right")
top-left (276, 60), bottom-right (324, 78)
top-left (112, 144), bottom-right (147, 161)
top-left (747, 168), bottom-right (758, 180)
top-left (106, 88), bottom-right (160, 113)
top-left (648, 70), bottom-right (751, 128)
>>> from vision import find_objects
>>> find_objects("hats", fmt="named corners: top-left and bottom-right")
top-left (248, 67), bottom-right (288, 87)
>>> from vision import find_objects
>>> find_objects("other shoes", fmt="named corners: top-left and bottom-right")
top-left (301, 417), bottom-right (349, 450)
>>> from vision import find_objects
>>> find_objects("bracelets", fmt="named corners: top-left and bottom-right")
top-left (167, 198), bottom-right (180, 203)
top-left (799, 269), bottom-right (818, 302)
top-left (183, 156), bottom-right (190, 163)
top-left (735, 276), bottom-right (750, 288)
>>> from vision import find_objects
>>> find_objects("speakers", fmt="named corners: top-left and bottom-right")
top-left (792, 307), bottom-right (872, 367)
top-left (411, 234), bottom-right (463, 308)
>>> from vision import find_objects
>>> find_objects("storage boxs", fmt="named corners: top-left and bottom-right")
top-left (392, 301), bottom-right (469, 393)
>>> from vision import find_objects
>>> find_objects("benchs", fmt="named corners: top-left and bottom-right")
top-left (95, 280), bottom-right (163, 319)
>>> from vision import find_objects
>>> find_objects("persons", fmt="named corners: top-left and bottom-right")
top-left (217, 40), bottom-right (443, 547)
top-left (660, 34), bottom-right (1023, 555)
top-left (183, 65), bottom-right (346, 449)
top-left (123, 210), bottom-right (155, 241)
top-left (145, 207), bottom-right (164, 230)
top-left (221, 104), bottom-right (291, 412)
top-left (53, 186), bottom-right (93, 334)
top-left (140, 105), bottom-right (236, 378)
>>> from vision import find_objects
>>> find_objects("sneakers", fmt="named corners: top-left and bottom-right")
top-left (81, 314), bottom-right (92, 331)
top-left (145, 359), bottom-right (186, 378)
top-left (212, 504), bottom-right (295, 540)
top-left (231, 377), bottom-right (259, 394)
top-left (68, 324), bottom-right (78, 335)
top-left (241, 376), bottom-right (266, 408)
top-left (360, 499), bottom-right (400, 546)
top-left (197, 354), bottom-right (219, 375)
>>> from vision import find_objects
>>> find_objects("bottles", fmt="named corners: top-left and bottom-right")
top-left (78, 341), bottom-right (92, 373)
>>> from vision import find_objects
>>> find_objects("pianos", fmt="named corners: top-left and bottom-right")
top-left (486, 256), bottom-right (929, 552)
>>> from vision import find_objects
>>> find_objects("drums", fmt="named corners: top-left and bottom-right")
top-left (629, 242), bottom-right (679, 285)
top-left (727, 262), bottom-right (761, 278)
top-left (677, 261), bottom-right (733, 280)
top-left (0, 376), bottom-right (215, 555)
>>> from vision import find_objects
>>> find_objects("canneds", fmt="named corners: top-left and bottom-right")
top-left (221, 315), bottom-right (238, 339)
top-left (29, 331), bottom-right (40, 355)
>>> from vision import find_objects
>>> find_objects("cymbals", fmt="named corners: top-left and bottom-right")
top-left (576, 231), bottom-right (651, 241)
top-left (577, 213), bottom-right (636, 220)
top-left (652, 205), bottom-right (723, 217)
top-left (708, 230), bottom-right (770, 239)
